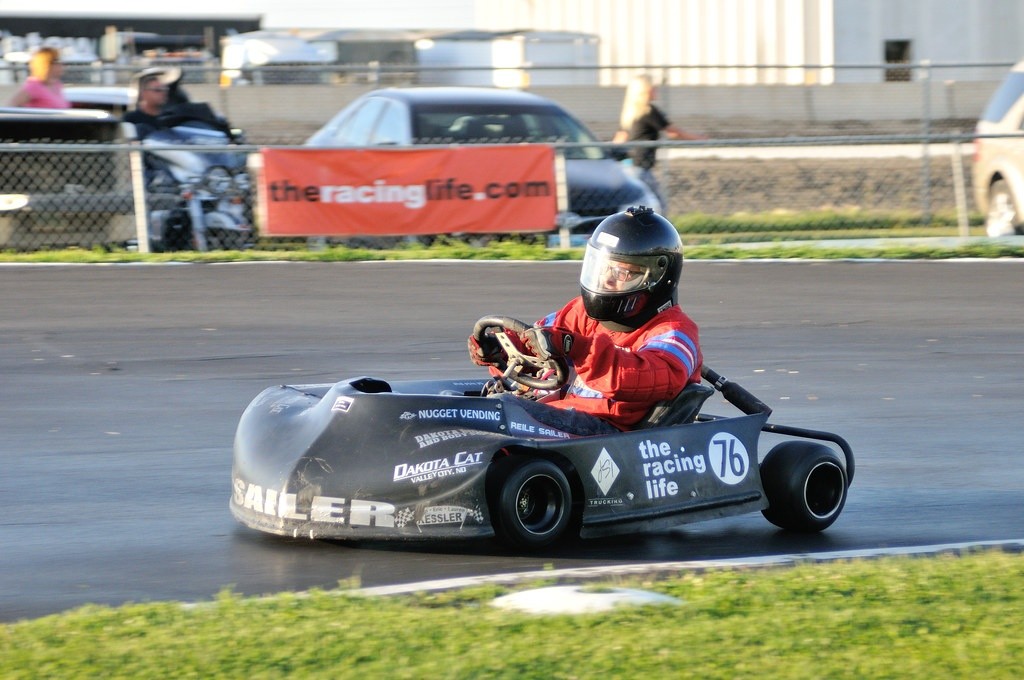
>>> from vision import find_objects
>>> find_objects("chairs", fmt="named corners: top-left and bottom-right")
top-left (632, 380), bottom-right (717, 432)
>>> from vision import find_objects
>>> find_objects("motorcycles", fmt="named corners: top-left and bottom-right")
top-left (118, 121), bottom-right (254, 252)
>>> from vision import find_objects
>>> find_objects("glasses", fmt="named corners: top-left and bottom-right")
top-left (597, 261), bottom-right (645, 283)
top-left (145, 86), bottom-right (170, 92)
top-left (50, 59), bottom-right (62, 65)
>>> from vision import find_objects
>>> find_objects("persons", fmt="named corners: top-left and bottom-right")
top-left (614, 74), bottom-right (708, 213)
top-left (12, 46), bottom-right (70, 110)
top-left (119, 80), bottom-right (171, 182)
top-left (98, 24), bottom-right (122, 80)
top-left (468, 207), bottom-right (703, 439)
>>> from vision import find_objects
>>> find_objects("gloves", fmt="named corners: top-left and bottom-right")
top-left (520, 326), bottom-right (589, 360)
top-left (468, 327), bottom-right (533, 368)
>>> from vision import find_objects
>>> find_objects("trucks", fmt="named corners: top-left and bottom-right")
top-left (221, 37), bottom-right (339, 89)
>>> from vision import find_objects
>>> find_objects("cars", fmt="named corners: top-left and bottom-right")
top-left (298, 87), bottom-right (664, 253)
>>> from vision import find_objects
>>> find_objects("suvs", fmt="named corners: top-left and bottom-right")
top-left (969, 58), bottom-right (1024, 245)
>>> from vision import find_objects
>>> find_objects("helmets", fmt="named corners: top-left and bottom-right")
top-left (580, 205), bottom-right (683, 321)
